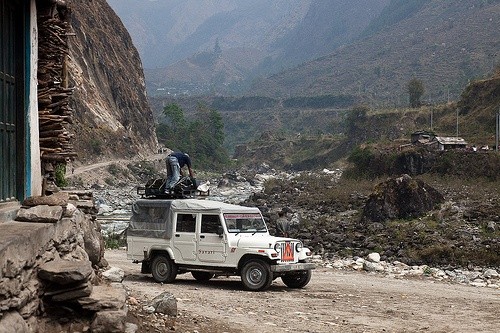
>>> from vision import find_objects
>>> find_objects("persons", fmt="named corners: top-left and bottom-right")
top-left (164, 150), bottom-right (195, 193)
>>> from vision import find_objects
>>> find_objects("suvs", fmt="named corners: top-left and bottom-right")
top-left (128, 188), bottom-right (316, 292)
